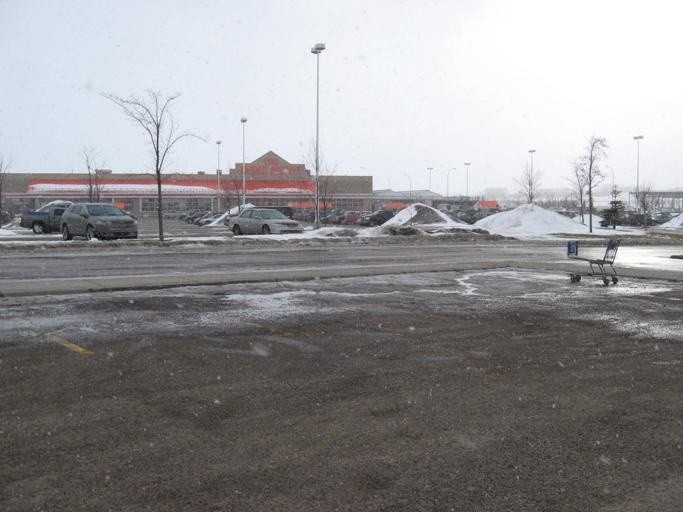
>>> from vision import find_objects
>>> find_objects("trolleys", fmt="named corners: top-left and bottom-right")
top-left (567, 238), bottom-right (621, 286)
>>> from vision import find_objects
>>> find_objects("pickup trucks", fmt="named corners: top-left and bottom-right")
top-left (20, 201), bottom-right (73, 235)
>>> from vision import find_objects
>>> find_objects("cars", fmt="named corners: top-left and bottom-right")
top-left (60, 203), bottom-right (138, 240)
top-left (229, 208), bottom-right (304, 235)
top-left (163, 208), bottom-right (225, 226)
top-left (438, 208), bottom-right (506, 224)
top-left (225, 206), bottom-right (293, 227)
top-left (646, 211), bottom-right (672, 227)
top-left (296, 208), bottom-right (394, 226)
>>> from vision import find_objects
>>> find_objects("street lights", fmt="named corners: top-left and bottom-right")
top-left (240, 117), bottom-right (247, 209)
top-left (311, 42), bottom-right (325, 230)
top-left (427, 167), bottom-right (433, 191)
top-left (216, 140), bottom-right (222, 215)
top-left (446, 167), bottom-right (457, 198)
top-left (402, 174), bottom-right (411, 197)
top-left (465, 163), bottom-right (472, 196)
top-left (632, 136), bottom-right (644, 212)
top-left (528, 150), bottom-right (535, 204)
